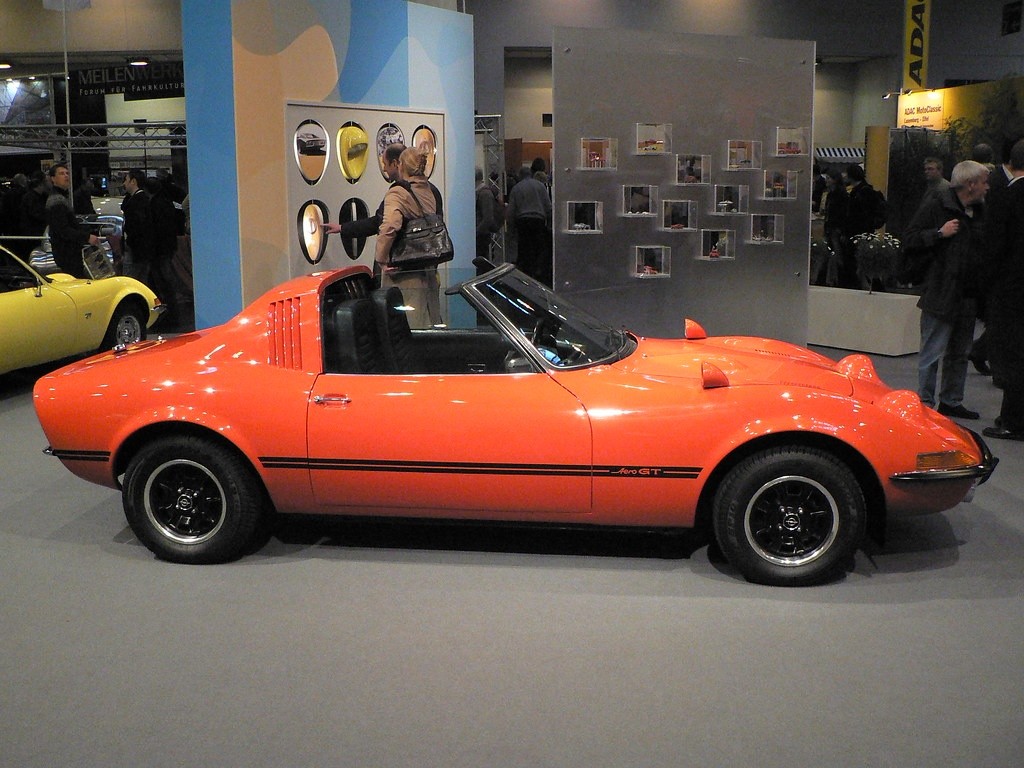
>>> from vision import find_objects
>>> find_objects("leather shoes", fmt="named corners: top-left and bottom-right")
top-left (995, 416), bottom-right (1002, 426)
top-left (983, 425), bottom-right (1024, 441)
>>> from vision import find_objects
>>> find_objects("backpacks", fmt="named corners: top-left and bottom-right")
top-left (478, 186), bottom-right (506, 233)
top-left (859, 185), bottom-right (890, 228)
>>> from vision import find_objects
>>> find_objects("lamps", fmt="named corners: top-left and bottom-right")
top-left (882, 92), bottom-right (902, 100)
top-left (904, 88), bottom-right (934, 95)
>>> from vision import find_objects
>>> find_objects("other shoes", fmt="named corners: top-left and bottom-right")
top-left (973, 357), bottom-right (990, 375)
top-left (937, 402), bottom-right (978, 419)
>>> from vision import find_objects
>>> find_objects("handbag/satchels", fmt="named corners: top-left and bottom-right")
top-left (389, 186), bottom-right (455, 270)
top-left (899, 211), bottom-right (949, 287)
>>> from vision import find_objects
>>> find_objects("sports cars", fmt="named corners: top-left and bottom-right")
top-left (32, 256), bottom-right (999, 586)
top-left (0, 244), bottom-right (168, 393)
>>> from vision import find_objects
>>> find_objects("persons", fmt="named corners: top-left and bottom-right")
top-left (121, 169), bottom-right (194, 314)
top-left (902, 139), bottom-right (1024, 440)
top-left (321, 144), bottom-right (454, 329)
top-left (812, 165), bottom-right (885, 288)
top-left (475, 158), bottom-right (552, 286)
top-left (0, 164), bottom-right (109, 279)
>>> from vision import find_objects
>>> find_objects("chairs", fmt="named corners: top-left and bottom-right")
top-left (373, 285), bottom-right (464, 374)
top-left (333, 298), bottom-right (400, 374)
